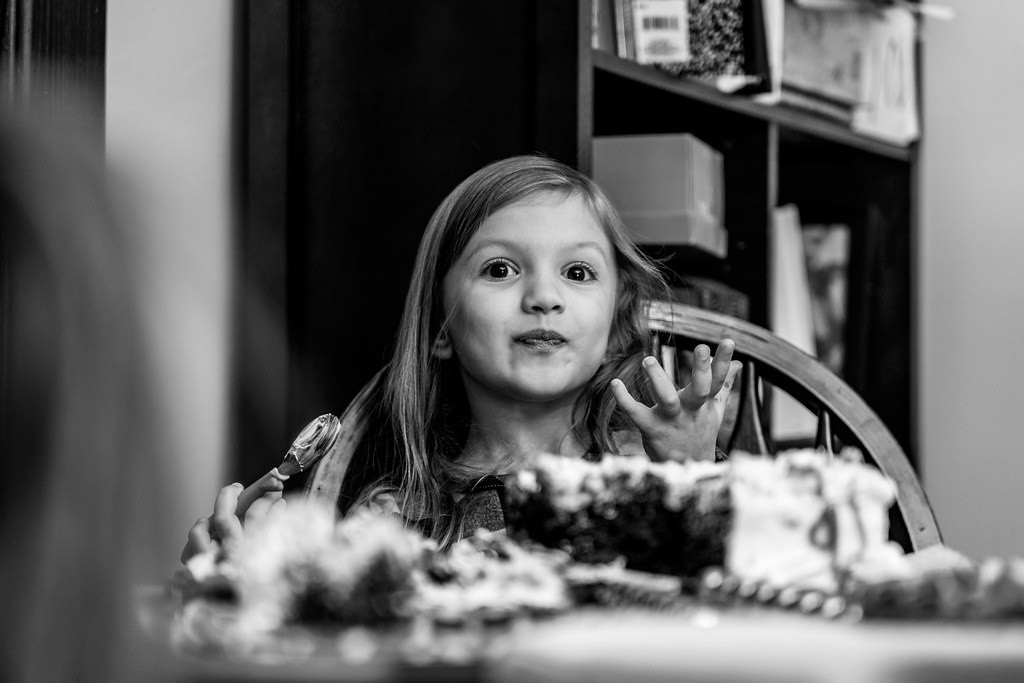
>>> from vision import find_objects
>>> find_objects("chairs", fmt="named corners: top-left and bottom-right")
top-left (311, 287), bottom-right (955, 616)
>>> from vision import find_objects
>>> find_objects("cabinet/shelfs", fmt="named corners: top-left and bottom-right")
top-left (242, 0), bottom-right (930, 532)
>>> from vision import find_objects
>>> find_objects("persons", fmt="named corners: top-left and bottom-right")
top-left (177, 154), bottom-right (747, 568)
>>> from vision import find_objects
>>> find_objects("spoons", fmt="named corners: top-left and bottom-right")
top-left (180, 414), bottom-right (342, 563)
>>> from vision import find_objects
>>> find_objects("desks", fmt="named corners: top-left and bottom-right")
top-left (115, 580), bottom-right (1024, 681)
